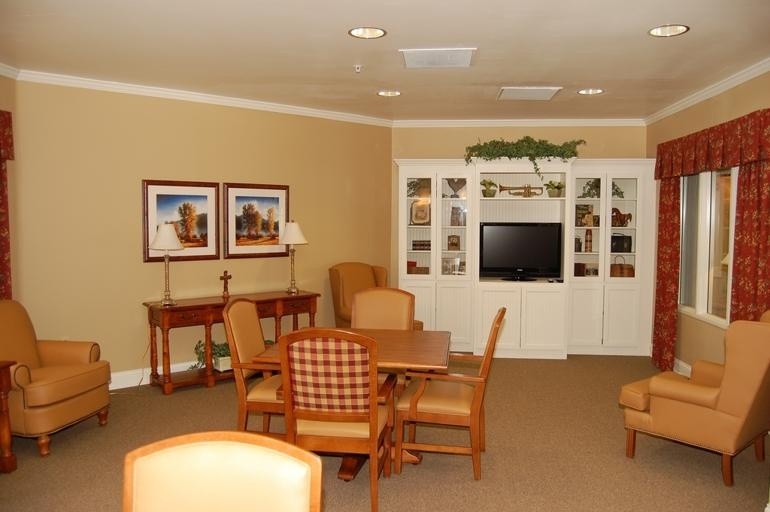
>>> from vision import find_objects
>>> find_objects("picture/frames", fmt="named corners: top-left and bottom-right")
top-left (141, 179), bottom-right (220, 261)
top-left (222, 182), bottom-right (289, 260)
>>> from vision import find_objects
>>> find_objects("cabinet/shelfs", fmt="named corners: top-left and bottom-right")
top-left (574, 166), bottom-right (645, 283)
top-left (398, 167), bottom-right (474, 281)
top-left (570, 285), bottom-right (643, 356)
top-left (400, 278), bottom-right (474, 354)
top-left (479, 287), bottom-right (568, 359)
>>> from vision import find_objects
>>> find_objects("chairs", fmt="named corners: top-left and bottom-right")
top-left (221, 297), bottom-right (288, 433)
top-left (121, 432), bottom-right (324, 512)
top-left (277, 327), bottom-right (397, 512)
top-left (0, 299), bottom-right (111, 457)
top-left (618, 309), bottom-right (770, 487)
top-left (351, 290), bottom-right (415, 330)
top-left (394, 307), bottom-right (505, 480)
top-left (328, 262), bottom-right (423, 332)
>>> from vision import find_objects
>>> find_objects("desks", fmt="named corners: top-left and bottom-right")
top-left (0, 361), bottom-right (18, 472)
top-left (142, 291), bottom-right (321, 395)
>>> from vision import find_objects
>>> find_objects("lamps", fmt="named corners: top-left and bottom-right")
top-left (279, 222), bottom-right (310, 293)
top-left (148, 223), bottom-right (184, 306)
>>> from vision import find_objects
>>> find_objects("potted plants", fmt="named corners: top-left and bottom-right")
top-left (543, 180), bottom-right (567, 197)
top-left (188, 340), bottom-right (273, 372)
top-left (480, 179), bottom-right (498, 197)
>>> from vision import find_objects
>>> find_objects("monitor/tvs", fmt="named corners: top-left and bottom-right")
top-left (479, 222), bottom-right (562, 281)
